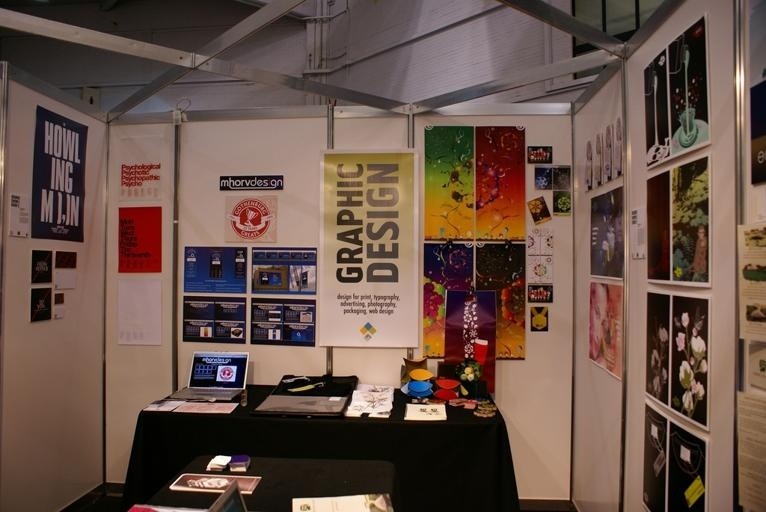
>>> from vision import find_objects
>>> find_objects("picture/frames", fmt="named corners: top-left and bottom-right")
top-left (208, 479), bottom-right (248, 512)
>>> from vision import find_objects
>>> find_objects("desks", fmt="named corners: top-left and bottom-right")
top-left (146, 453), bottom-right (396, 511)
top-left (121, 383), bottom-right (522, 511)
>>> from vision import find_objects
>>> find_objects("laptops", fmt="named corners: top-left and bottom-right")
top-left (169, 350), bottom-right (251, 401)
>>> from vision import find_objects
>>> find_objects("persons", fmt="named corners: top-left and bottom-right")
top-left (590, 283), bottom-right (610, 359)
